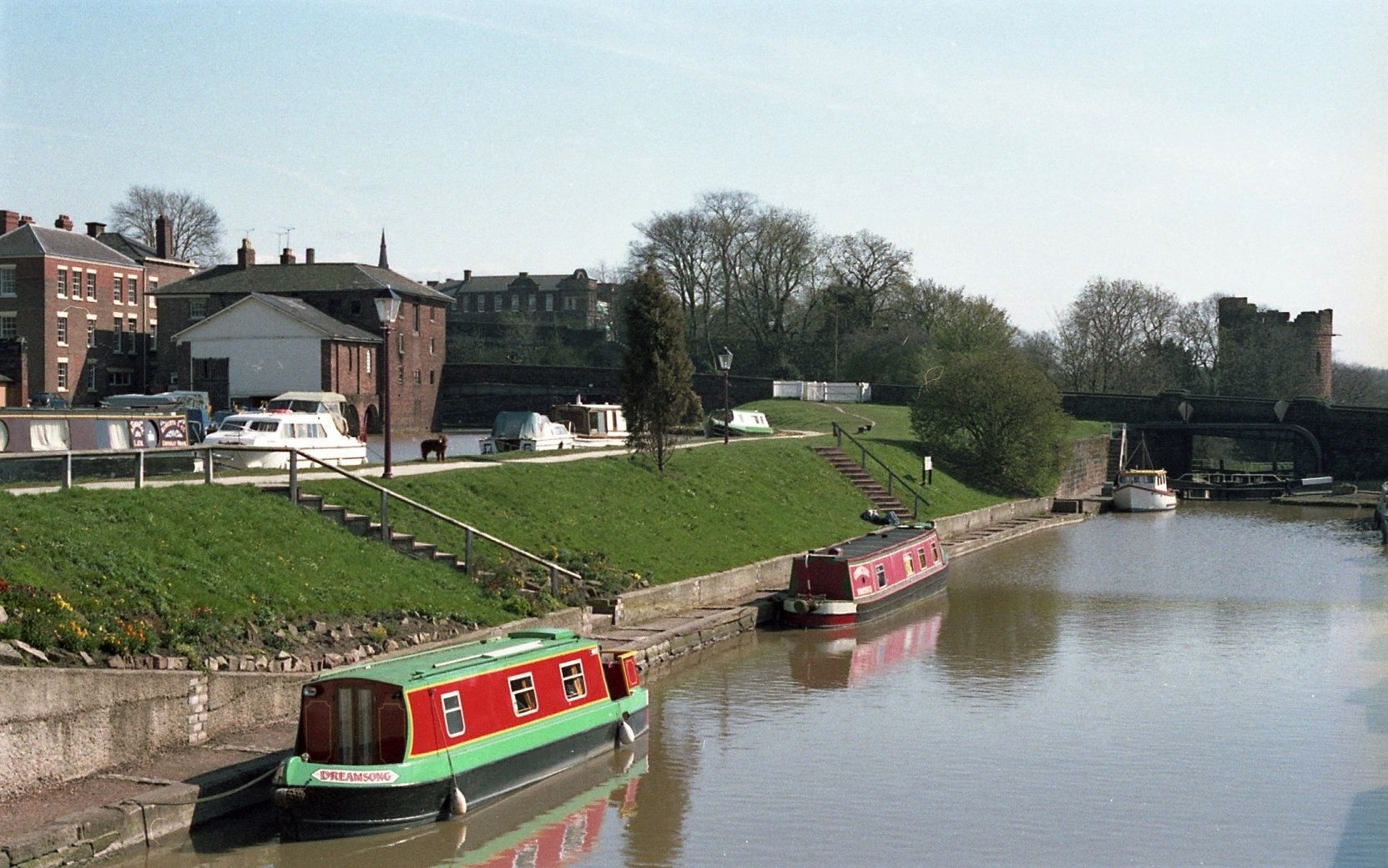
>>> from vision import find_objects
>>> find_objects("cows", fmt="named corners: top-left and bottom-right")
top-left (421, 434), bottom-right (448, 462)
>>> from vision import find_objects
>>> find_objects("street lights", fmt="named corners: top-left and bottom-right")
top-left (373, 284), bottom-right (402, 481)
top-left (717, 345), bottom-right (733, 445)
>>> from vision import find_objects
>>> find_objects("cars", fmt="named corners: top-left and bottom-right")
top-left (27, 391), bottom-right (69, 409)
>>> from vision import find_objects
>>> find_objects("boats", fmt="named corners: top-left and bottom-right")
top-left (1164, 470), bottom-right (1295, 502)
top-left (1110, 429), bottom-right (1179, 513)
top-left (268, 626), bottom-right (651, 844)
top-left (0, 406), bottom-right (193, 481)
top-left (192, 389), bottom-right (370, 474)
top-left (1374, 480), bottom-right (1388, 545)
top-left (774, 516), bottom-right (950, 630)
top-left (707, 408), bottom-right (774, 438)
top-left (550, 394), bottom-right (653, 448)
top-left (477, 410), bottom-right (578, 454)
